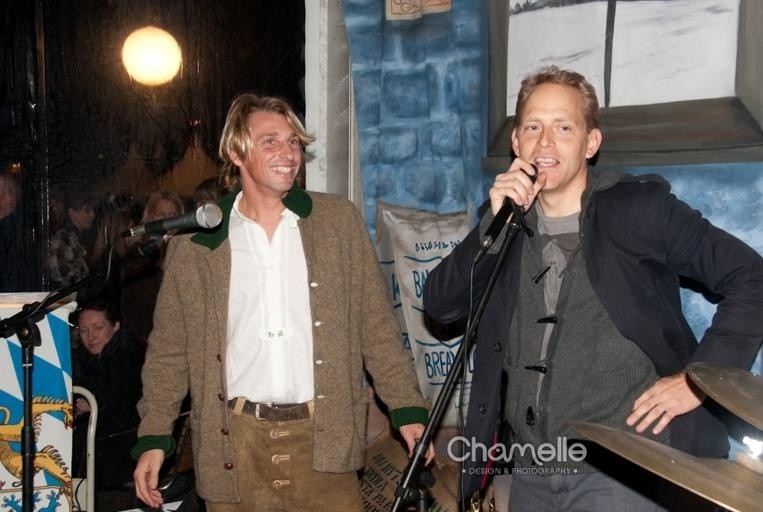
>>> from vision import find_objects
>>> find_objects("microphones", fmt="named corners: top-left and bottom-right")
top-left (479, 163), bottom-right (538, 251)
top-left (129, 202), bottom-right (222, 238)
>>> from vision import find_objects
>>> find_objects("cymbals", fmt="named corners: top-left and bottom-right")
top-left (568, 419), bottom-right (762, 512)
top-left (687, 361), bottom-right (763, 432)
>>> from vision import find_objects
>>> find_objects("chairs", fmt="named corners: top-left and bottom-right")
top-left (71, 406), bottom-right (194, 495)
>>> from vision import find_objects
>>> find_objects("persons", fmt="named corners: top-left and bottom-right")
top-left (2, 160), bottom-right (248, 290)
top-left (420, 66), bottom-right (762, 512)
top-left (133, 94), bottom-right (437, 511)
top-left (71, 302), bottom-right (142, 511)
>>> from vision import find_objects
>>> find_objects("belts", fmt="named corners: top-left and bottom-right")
top-left (228, 398), bottom-right (309, 421)
